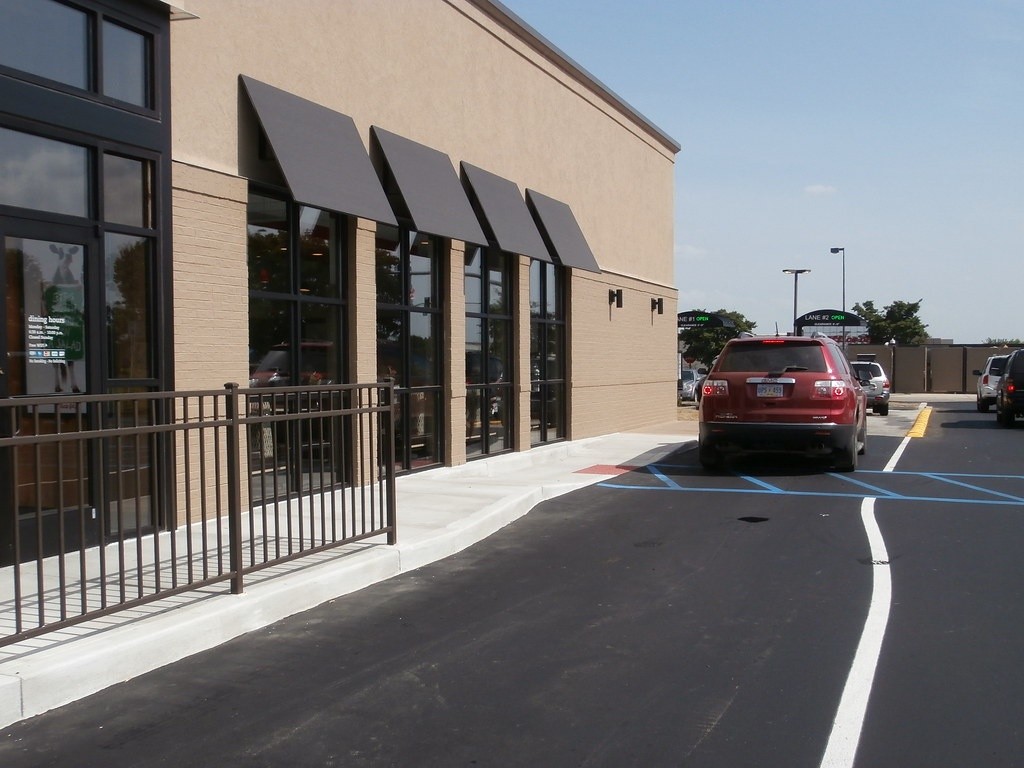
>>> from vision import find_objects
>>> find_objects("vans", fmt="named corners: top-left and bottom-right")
top-left (682, 368), bottom-right (701, 401)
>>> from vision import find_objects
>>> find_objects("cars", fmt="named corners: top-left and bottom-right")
top-left (695, 354), bottom-right (720, 410)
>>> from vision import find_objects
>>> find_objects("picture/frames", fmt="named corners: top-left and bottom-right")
top-left (17, 237), bottom-right (115, 420)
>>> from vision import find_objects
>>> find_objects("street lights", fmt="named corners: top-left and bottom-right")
top-left (782, 269), bottom-right (811, 336)
top-left (831, 247), bottom-right (845, 352)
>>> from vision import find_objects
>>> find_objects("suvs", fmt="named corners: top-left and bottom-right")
top-left (973, 353), bottom-right (1010, 412)
top-left (698, 323), bottom-right (868, 472)
top-left (850, 361), bottom-right (890, 416)
top-left (997, 347), bottom-right (1024, 426)
